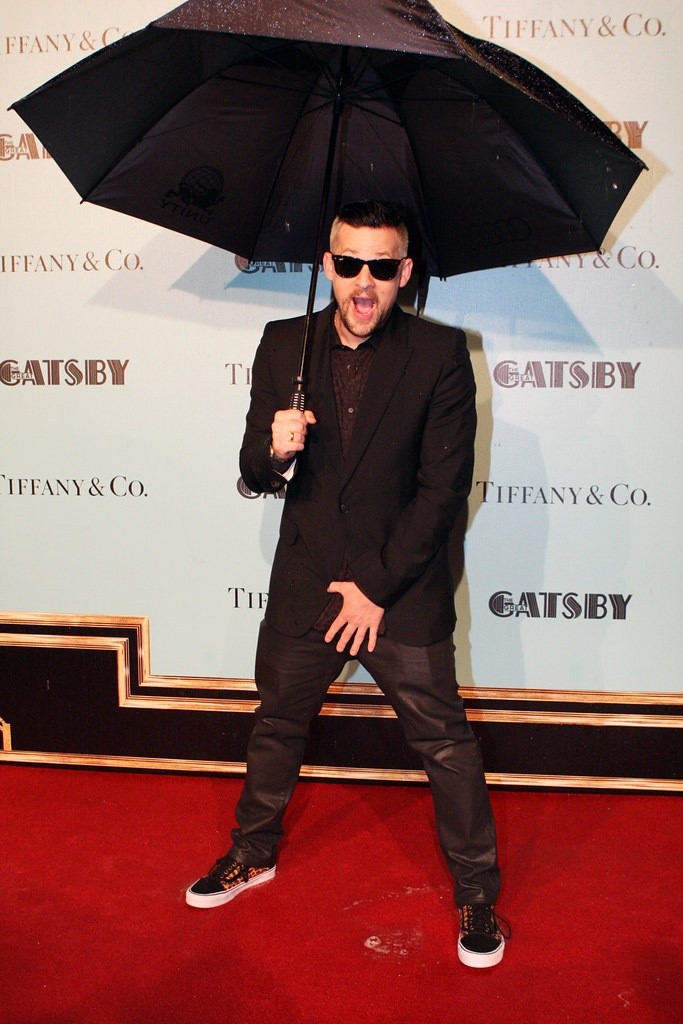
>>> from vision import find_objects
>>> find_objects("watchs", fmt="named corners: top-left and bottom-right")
top-left (268, 445), bottom-right (288, 463)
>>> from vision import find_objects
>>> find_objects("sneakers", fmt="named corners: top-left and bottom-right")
top-left (457, 902), bottom-right (512, 969)
top-left (185, 856), bottom-right (277, 909)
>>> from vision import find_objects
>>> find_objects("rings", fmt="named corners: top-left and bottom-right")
top-left (290, 432), bottom-right (294, 442)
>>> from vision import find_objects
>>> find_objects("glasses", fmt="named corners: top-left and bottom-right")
top-left (330, 251), bottom-right (407, 282)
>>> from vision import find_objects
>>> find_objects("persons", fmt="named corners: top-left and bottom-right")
top-left (184, 201), bottom-right (510, 968)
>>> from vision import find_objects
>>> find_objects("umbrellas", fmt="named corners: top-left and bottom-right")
top-left (7, 0), bottom-right (651, 412)
